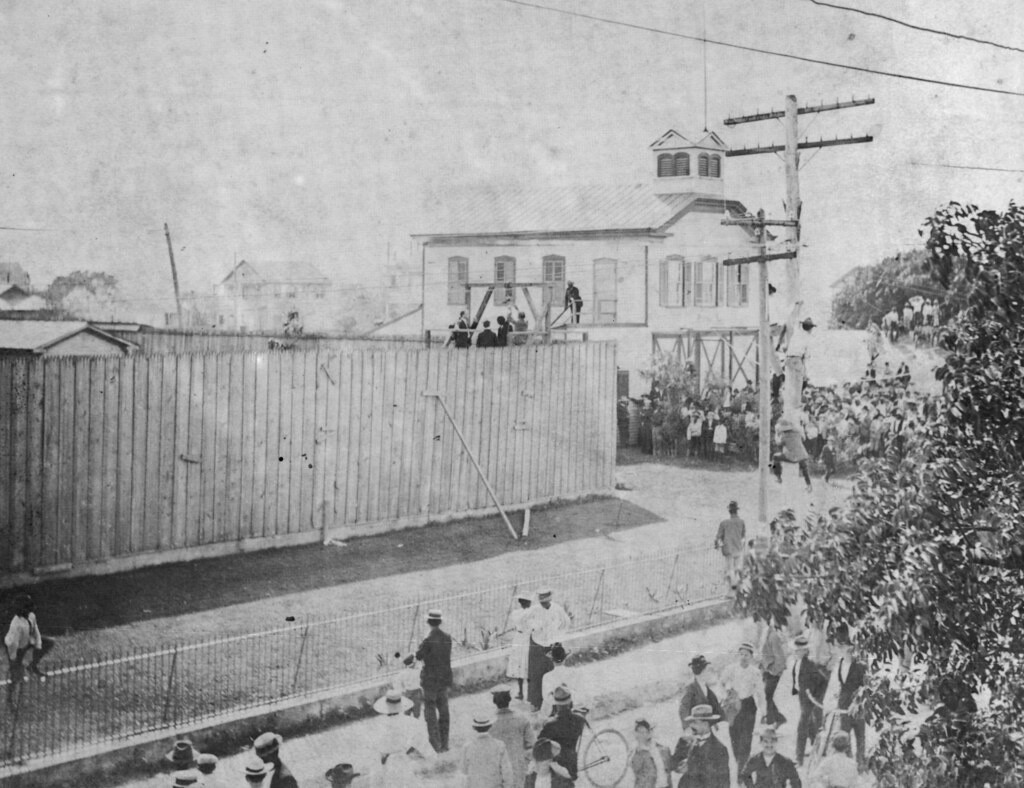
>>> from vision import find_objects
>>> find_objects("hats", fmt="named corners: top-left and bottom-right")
top-left (685, 705), bottom-right (720, 720)
top-left (688, 655), bottom-right (711, 666)
top-left (490, 685), bottom-right (511, 694)
top-left (374, 690), bottom-right (414, 713)
top-left (514, 591), bottom-right (531, 601)
top-left (536, 589), bottom-right (552, 602)
top-left (739, 642), bottom-right (753, 652)
top-left (548, 686), bottom-right (571, 705)
top-left (471, 713), bottom-right (497, 726)
top-left (755, 727), bottom-right (783, 737)
top-left (425, 610), bottom-right (441, 619)
top-left (524, 737), bottom-right (561, 760)
top-left (197, 754), bottom-right (218, 764)
top-left (254, 732), bottom-right (282, 757)
top-left (403, 654), bottom-right (414, 667)
top-left (727, 500), bottom-right (740, 512)
top-left (243, 758), bottom-right (274, 774)
top-left (172, 773), bottom-right (197, 787)
top-left (325, 764), bottom-right (360, 782)
top-left (165, 741), bottom-right (199, 762)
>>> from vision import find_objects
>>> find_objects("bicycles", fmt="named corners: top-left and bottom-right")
top-left (566, 707), bottom-right (629, 788)
top-left (806, 688), bottom-right (852, 782)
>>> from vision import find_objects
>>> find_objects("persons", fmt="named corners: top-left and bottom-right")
top-left (372, 609), bottom-right (453, 788)
top-left (164, 740), bottom-right (218, 788)
top-left (632, 614), bottom-right (868, 788)
top-left (565, 280), bottom-right (583, 323)
top-left (456, 311), bottom-right (528, 347)
top-left (715, 501), bottom-right (745, 590)
top-left (506, 590), bottom-right (574, 723)
top-left (885, 290), bottom-right (953, 343)
top-left (239, 732), bottom-right (299, 788)
top-left (617, 357), bottom-right (911, 491)
top-left (461, 685), bottom-right (585, 788)
top-left (5, 594), bottom-right (54, 710)
top-left (325, 764), bottom-right (360, 788)
top-left (787, 317), bottom-right (816, 388)
